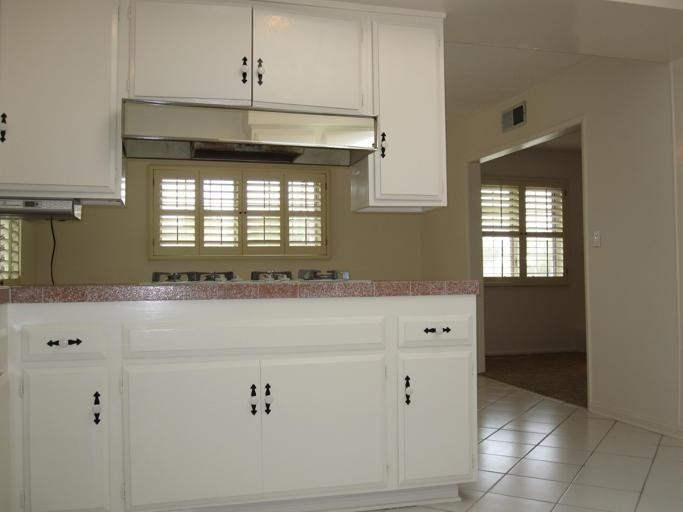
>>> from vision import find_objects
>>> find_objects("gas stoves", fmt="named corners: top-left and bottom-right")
top-left (151, 268), bottom-right (350, 282)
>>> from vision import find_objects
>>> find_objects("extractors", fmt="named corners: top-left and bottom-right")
top-left (123, 99), bottom-right (377, 168)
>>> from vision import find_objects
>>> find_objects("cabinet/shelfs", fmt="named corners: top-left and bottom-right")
top-left (1, 1), bottom-right (126, 206)
top-left (122, 298), bottom-right (391, 512)
top-left (121, 1), bottom-right (372, 118)
top-left (1, 301), bottom-right (125, 512)
top-left (349, 11), bottom-right (448, 211)
top-left (390, 294), bottom-right (479, 508)
